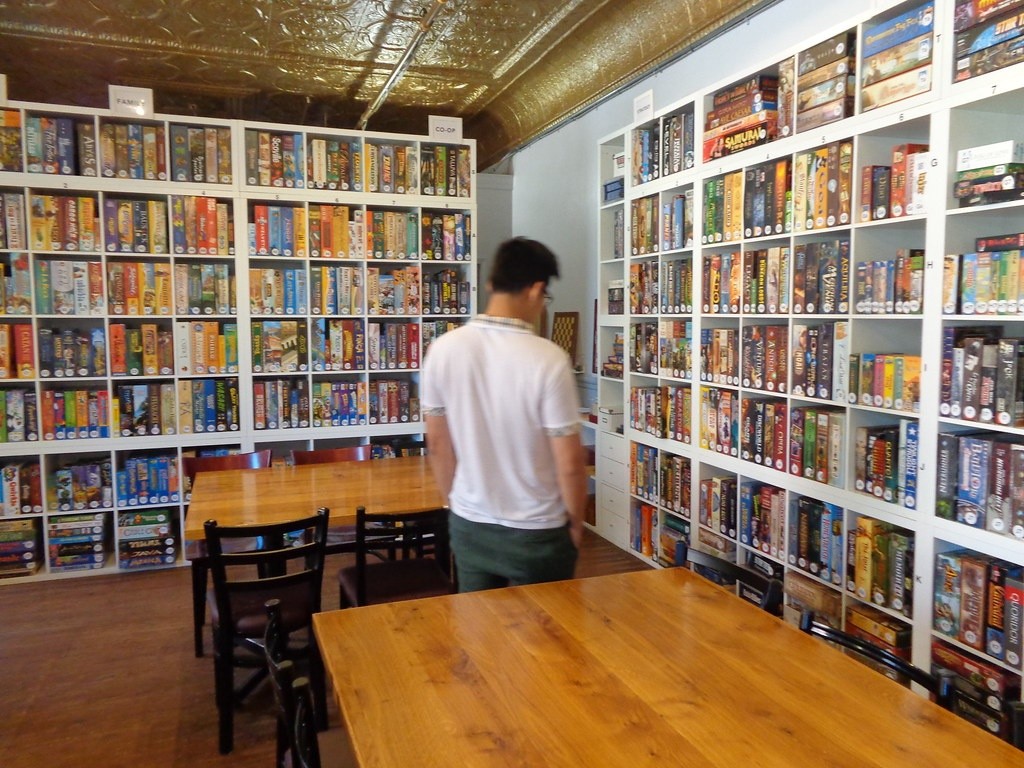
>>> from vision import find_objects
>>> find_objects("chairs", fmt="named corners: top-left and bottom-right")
top-left (798, 607), bottom-right (958, 713)
top-left (182, 448), bottom-right (294, 659)
top-left (290, 677), bottom-right (322, 768)
top-left (262, 599), bottom-right (383, 768)
top-left (205, 507), bottom-right (330, 756)
top-left (290, 443), bottom-right (400, 571)
top-left (337, 504), bottom-right (457, 610)
top-left (387, 441), bottom-right (442, 559)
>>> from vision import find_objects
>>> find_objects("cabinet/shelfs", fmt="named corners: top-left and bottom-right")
top-left (580, 419), bottom-right (597, 532)
top-left (595, 0), bottom-right (1024, 701)
top-left (0, 98), bottom-right (477, 587)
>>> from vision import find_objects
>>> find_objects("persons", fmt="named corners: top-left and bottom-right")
top-left (419, 236), bottom-right (585, 591)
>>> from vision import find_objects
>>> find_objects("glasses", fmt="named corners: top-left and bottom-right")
top-left (542, 292), bottom-right (553, 306)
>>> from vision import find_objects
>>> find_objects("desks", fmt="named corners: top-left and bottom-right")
top-left (184, 455), bottom-right (447, 543)
top-left (311, 566), bottom-right (1024, 768)
